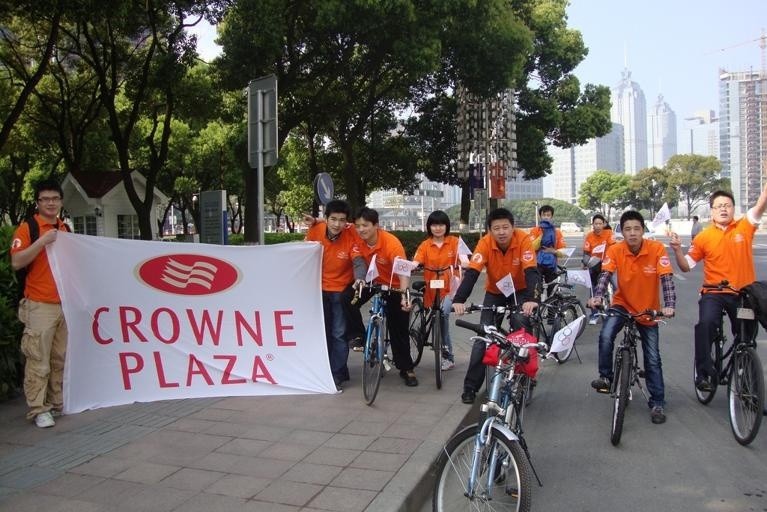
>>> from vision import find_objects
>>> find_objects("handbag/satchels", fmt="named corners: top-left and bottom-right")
top-left (450, 274), bottom-right (462, 299)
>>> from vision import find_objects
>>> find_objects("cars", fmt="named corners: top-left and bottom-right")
top-left (613, 224), bottom-right (656, 244)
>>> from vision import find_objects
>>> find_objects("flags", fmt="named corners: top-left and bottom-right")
top-left (392, 256), bottom-right (417, 277)
top-left (560, 247), bottom-right (574, 255)
top-left (592, 244), bottom-right (605, 253)
top-left (496, 274), bottom-right (516, 297)
top-left (365, 253), bottom-right (379, 284)
top-left (550, 316), bottom-right (581, 352)
top-left (457, 238), bottom-right (472, 256)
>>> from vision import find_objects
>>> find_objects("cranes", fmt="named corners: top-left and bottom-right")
top-left (722, 28), bottom-right (767, 74)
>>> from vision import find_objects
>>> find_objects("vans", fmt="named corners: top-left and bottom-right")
top-left (560, 222), bottom-right (584, 234)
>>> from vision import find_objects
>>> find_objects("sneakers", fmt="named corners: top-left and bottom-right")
top-left (461, 390), bottom-right (476, 402)
top-left (400, 370), bottom-right (418, 386)
top-left (652, 406), bottom-right (665, 424)
top-left (592, 376), bottom-right (611, 389)
top-left (442, 359), bottom-right (455, 371)
top-left (589, 316), bottom-right (600, 324)
top-left (696, 376), bottom-right (708, 388)
top-left (34, 412), bottom-right (56, 427)
top-left (350, 338), bottom-right (363, 348)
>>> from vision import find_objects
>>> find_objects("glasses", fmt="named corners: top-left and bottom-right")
top-left (36, 197), bottom-right (63, 202)
top-left (712, 206), bottom-right (734, 210)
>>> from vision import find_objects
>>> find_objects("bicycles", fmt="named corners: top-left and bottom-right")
top-left (432, 317), bottom-right (560, 512)
top-left (451, 265), bottom-right (587, 363)
top-left (354, 278), bottom-right (410, 407)
top-left (582, 259), bottom-right (615, 322)
top-left (583, 301), bottom-right (674, 446)
top-left (403, 264), bottom-right (464, 390)
top-left (693, 279), bottom-right (766, 445)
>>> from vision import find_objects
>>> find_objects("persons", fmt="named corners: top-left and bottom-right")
top-left (529, 206), bottom-right (567, 324)
top-left (11, 183), bottom-right (72, 427)
top-left (690, 216), bottom-right (703, 245)
top-left (582, 214), bottom-right (617, 324)
top-left (302, 206), bottom-right (418, 386)
top-left (587, 210), bottom-right (676, 424)
top-left (670, 181), bottom-right (767, 415)
top-left (303, 199), bottom-right (366, 392)
top-left (451, 207), bottom-right (540, 404)
top-left (412, 210), bottom-right (470, 370)
